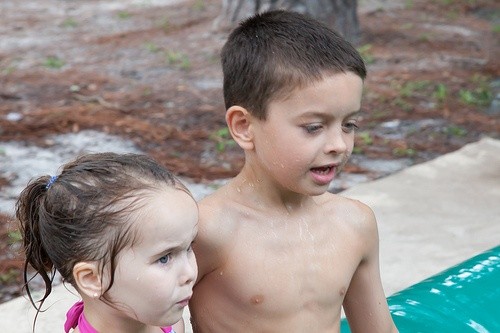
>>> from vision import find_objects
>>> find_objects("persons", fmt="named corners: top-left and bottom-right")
top-left (10, 149), bottom-right (200, 332)
top-left (182, 8), bottom-right (402, 332)
